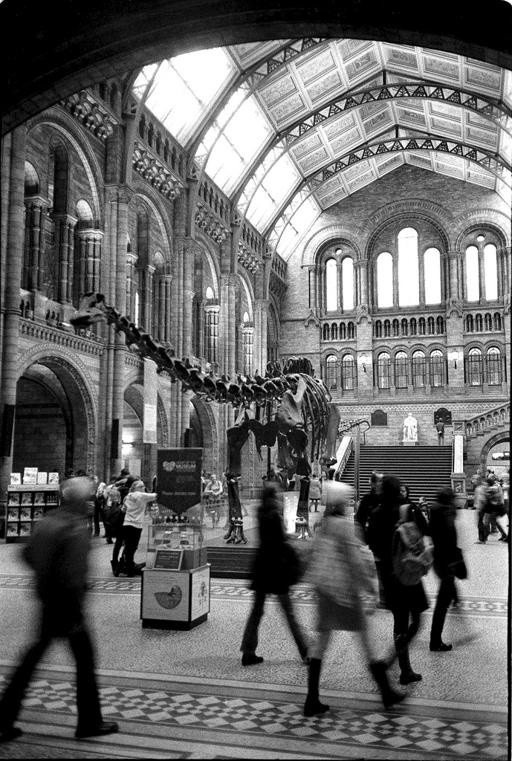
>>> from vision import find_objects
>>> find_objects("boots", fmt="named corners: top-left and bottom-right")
top-left (302, 657), bottom-right (331, 718)
top-left (366, 659), bottom-right (409, 712)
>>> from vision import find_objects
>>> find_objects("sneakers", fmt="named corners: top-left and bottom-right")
top-left (239, 650), bottom-right (264, 667)
top-left (109, 558), bottom-right (146, 577)
top-left (428, 644), bottom-right (454, 652)
top-left (400, 671), bottom-right (423, 686)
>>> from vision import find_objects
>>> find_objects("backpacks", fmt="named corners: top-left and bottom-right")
top-left (392, 503), bottom-right (436, 587)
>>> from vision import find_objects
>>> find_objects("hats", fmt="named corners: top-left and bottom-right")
top-left (321, 477), bottom-right (355, 505)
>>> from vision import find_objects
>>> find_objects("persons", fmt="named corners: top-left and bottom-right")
top-left (76, 468), bottom-right (158, 578)
top-left (0, 475), bottom-right (118, 743)
top-left (436, 418), bottom-right (444, 446)
top-left (403, 412), bottom-right (417, 440)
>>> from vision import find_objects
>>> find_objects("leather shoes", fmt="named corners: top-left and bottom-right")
top-left (74, 721), bottom-right (120, 738)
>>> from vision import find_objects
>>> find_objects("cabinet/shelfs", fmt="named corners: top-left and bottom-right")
top-left (5, 490), bottom-right (62, 544)
